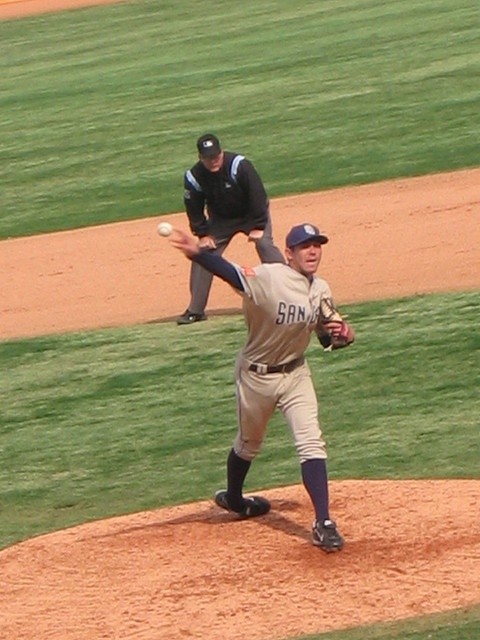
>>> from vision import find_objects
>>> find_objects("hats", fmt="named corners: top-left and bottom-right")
top-left (286, 224), bottom-right (327, 248)
top-left (197, 134), bottom-right (220, 158)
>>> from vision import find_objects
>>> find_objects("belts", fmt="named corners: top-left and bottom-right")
top-left (248, 356), bottom-right (304, 373)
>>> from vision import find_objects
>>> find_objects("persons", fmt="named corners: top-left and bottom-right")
top-left (176, 133), bottom-right (286, 323)
top-left (167, 222), bottom-right (354, 551)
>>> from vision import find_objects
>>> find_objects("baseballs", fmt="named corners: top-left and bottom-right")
top-left (157, 222), bottom-right (173, 237)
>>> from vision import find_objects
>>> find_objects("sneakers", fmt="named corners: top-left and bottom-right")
top-left (177, 309), bottom-right (207, 324)
top-left (312, 519), bottom-right (343, 547)
top-left (215, 490), bottom-right (270, 517)
